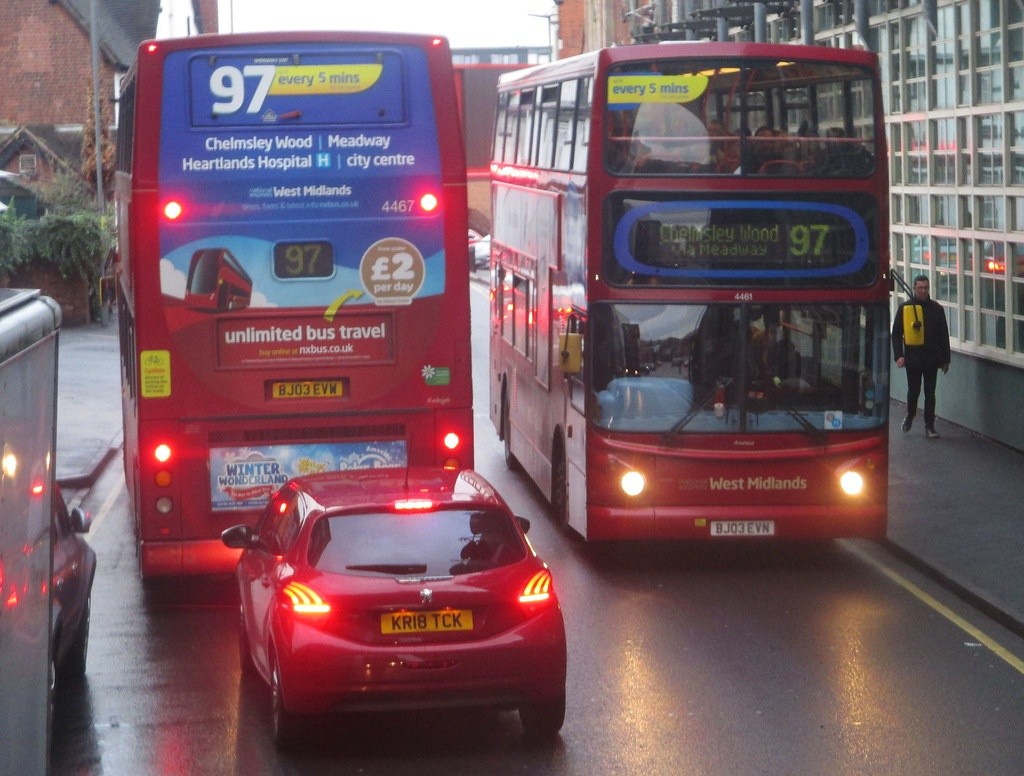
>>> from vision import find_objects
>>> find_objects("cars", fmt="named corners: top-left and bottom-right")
top-left (468, 230), bottom-right (492, 269)
top-left (218, 464), bottom-right (569, 741)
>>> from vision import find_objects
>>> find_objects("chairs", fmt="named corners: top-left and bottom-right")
top-left (594, 376), bottom-right (693, 432)
top-left (606, 109), bottom-right (867, 167)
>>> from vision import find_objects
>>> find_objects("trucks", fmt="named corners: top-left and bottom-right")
top-left (0, 287), bottom-right (97, 776)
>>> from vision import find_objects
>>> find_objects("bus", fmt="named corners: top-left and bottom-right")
top-left (96, 29), bottom-right (471, 582)
top-left (486, 39), bottom-right (926, 548)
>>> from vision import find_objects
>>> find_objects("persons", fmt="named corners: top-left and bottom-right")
top-left (892, 276), bottom-right (952, 439)
top-left (624, 111), bottom-right (871, 175)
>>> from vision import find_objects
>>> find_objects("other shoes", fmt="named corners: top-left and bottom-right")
top-left (925, 427), bottom-right (939, 437)
top-left (901, 411), bottom-right (917, 432)
